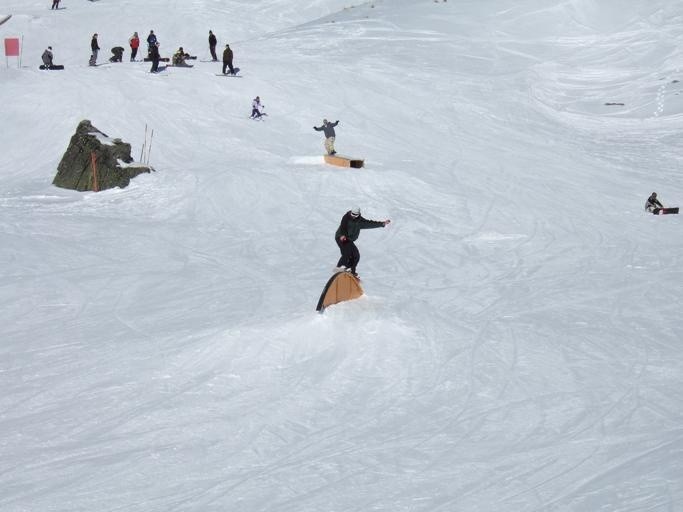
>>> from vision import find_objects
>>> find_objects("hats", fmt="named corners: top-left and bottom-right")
top-left (351, 207), bottom-right (360, 217)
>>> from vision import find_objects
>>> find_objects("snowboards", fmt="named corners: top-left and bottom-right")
top-left (330, 151), bottom-right (336, 155)
top-left (216, 74), bottom-right (242, 77)
top-left (39, 65), bottom-right (64, 70)
top-left (186, 57), bottom-right (196, 59)
top-left (653, 208), bottom-right (679, 214)
top-left (166, 65), bottom-right (193, 67)
top-left (200, 60), bottom-right (223, 62)
top-left (144, 58), bottom-right (170, 62)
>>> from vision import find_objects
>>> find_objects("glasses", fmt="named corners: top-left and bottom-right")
top-left (352, 213), bottom-right (359, 215)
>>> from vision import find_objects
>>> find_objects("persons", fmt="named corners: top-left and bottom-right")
top-left (335, 205), bottom-right (390, 276)
top-left (42, 46), bottom-right (53, 70)
top-left (644, 192), bottom-right (663, 213)
top-left (109, 47), bottom-right (124, 62)
top-left (209, 30), bottom-right (217, 61)
top-left (91, 34), bottom-right (100, 66)
top-left (222, 44), bottom-right (235, 76)
top-left (249, 96), bottom-right (262, 119)
top-left (313, 118), bottom-right (339, 155)
top-left (129, 30), bottom-right (191, 72)
top-left (52, 0), bottom-right (61, 9)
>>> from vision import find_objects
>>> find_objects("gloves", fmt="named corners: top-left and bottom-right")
top-left (340, 235), bottom-right (347, 242)
top-left (385, 220), bottom-right (390, 224)
